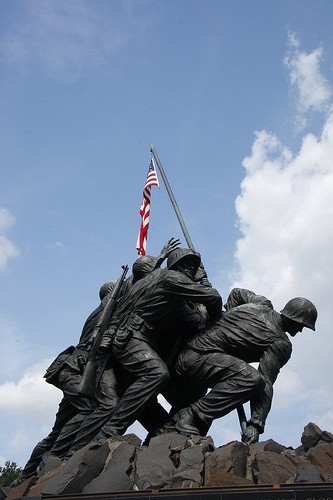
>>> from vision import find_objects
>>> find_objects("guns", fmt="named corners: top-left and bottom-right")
top-left (77, 264), bottom-right (129, 397)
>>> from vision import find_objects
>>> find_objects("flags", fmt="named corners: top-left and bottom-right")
top-left (136, 153), bottom-right (160, 256)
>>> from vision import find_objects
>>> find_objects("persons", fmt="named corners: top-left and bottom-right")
top-left (94, 247), bottom-right (222, 440)
top-left (10, 237), bottom-right (228, 488)
top-left (174, 288), bottom-right (317, 443)
